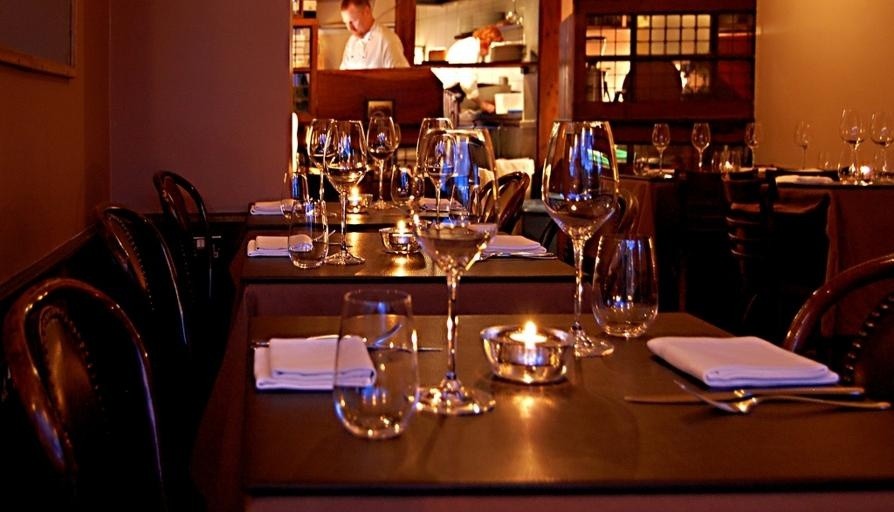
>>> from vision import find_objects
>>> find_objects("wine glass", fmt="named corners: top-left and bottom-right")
top-left (404, 115), bottom-right (504, 417)
top-left (690, 121), bottom-right (712, 173)
top-left (306, 112), bottom-right (402, 267)
top-left (792, 119), bottom-right (815, 173)
top-left (651, 122), bottom-right (672, 177)
top-left (837, 107), bottom-right (894, 187)
top-left (538, 118), bottom-right (621, 360)
top-left (744, 122), bottom-right (765, 169)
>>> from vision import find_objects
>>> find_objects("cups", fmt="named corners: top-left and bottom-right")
top-left (817, 149), bottom-right (841, 173)
top-left (632, 150), bottom-right (649, 178)
top-left (389, 161), bottom-right (417, 208)
top-left (279, 170), bottom-right (331, 271)
top-left (330, 286), bottom-right (422, 444)
top-left (587, 231), bottom-right (664, 342)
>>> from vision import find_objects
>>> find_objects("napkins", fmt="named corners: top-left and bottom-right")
top-left (422, 196), bottom-right (465, 213)
top-left (250, 196), bottom-right (302, 214)
top-left (480, 234), bottom-right (546, 260)
top-left (251, 330), bottom-right (376, 391)
top-left (648, 333), bottom-right (841, 389)
top-left (247, 234), bottom-right (314, 257)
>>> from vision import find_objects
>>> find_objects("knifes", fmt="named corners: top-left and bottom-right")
top-left (624, 385), bottom-right (866, 405)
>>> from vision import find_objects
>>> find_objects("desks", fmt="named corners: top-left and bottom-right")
top-left (197, 311), bottom-right (891, 511)
top-left (247, 195), bottom-right (473, 226)
top-left (232, 228), bottom-right (598, 313)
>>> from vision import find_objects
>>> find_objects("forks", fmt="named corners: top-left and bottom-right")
top-left (672, 378), bottom-right (891, 417)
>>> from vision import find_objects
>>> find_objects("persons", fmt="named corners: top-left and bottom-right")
top-left (436, 23), bottom-right (504, 126)
top-left (339, 0), bottom-right (410, 71)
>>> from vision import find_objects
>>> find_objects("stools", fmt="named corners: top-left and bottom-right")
top-left (721, 174), bottom-right (828, 338)
top-left (653, 174), bottom-right (719, 311)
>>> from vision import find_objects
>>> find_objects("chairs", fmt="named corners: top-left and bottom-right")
top-left (93, 198), bottom-right (229, 428)
top-left (783, 251), bottom-right (894, 401)
top-left (154, 172), bottom-right (237, 317)
top-left (1, 277), bottom-right (204, 504)
top-left (472, 172), bottom-right (530, 236)
top-left (545, 175), bottom-right (657, 298)
